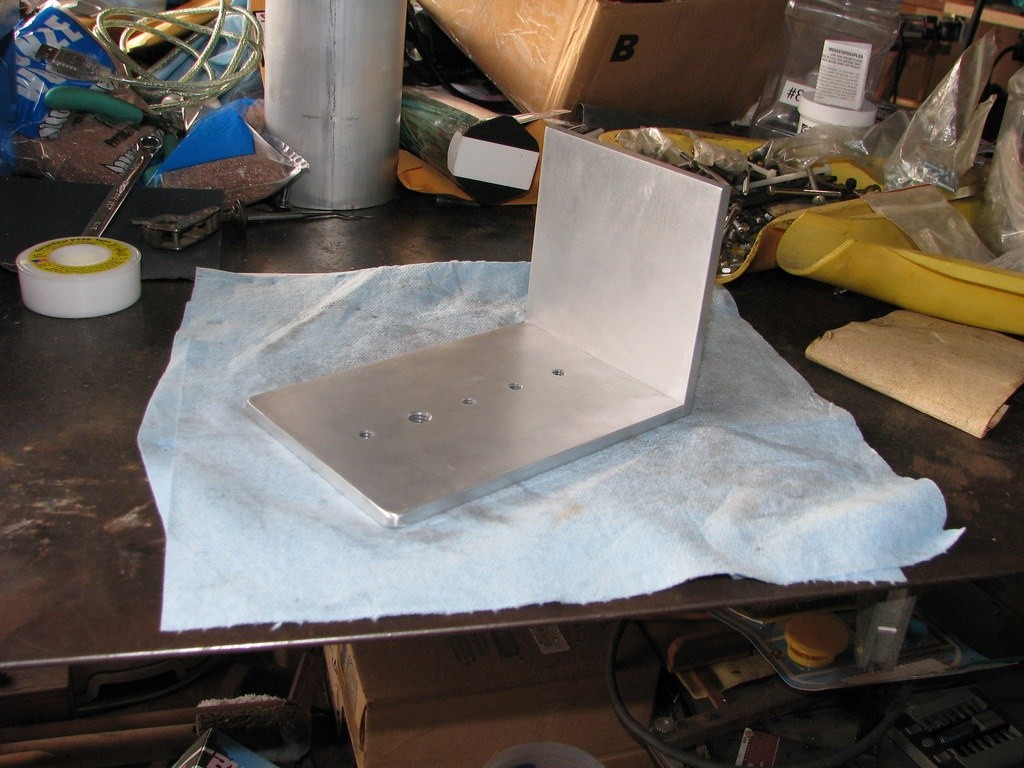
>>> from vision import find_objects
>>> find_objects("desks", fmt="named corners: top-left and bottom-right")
top-left (1, 177), bottom-right (1024, 674)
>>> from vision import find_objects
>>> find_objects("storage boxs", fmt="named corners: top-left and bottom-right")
top-left (418, 0), bottom-right (791, 132)
top-left (324, 626), bottom-right (681, 768)
top-left (784, 0), bottom-right (902, 103)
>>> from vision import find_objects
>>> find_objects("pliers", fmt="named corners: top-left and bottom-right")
top-left (44, 84), bottom-right (185, 183)
